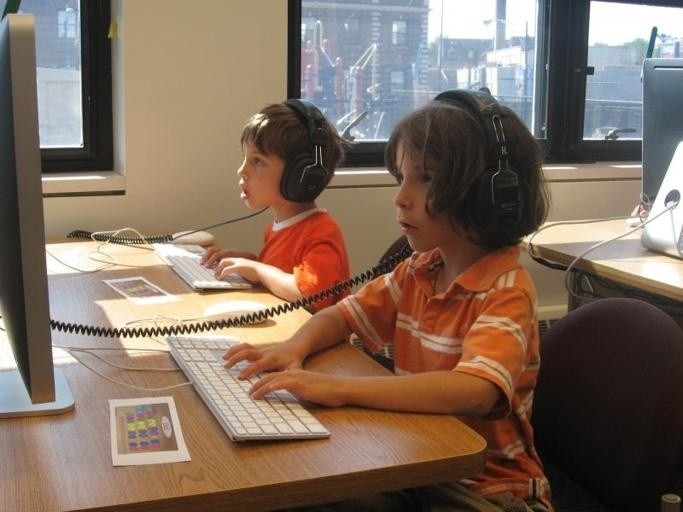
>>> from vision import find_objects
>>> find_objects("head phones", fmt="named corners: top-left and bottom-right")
top-left (279, 99), bottom-right (331, 204)
top-left (437, 87), bottom-right (528, 247)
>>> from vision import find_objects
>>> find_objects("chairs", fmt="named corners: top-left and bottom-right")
top-left (348, 235), bottom-right (412, 284)
top-left (531, 297), bottom-right (683, 511)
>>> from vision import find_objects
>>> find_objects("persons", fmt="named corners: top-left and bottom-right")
top-left (199, 96), bottom-right (353, 340)
top-left (220, 85), bottom-right (554, 511)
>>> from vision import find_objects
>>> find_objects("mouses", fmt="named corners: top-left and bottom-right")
top-left (169, 231), bottom-right (213, 246)
top-left (204, 301), bottom-right (269, 325)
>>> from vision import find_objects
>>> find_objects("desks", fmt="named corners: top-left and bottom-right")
top-left (0, 227), bottom-right (489, 512)
top-left (518, 214), bottom-right (683, 306)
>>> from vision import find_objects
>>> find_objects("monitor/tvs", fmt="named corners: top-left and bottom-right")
top-left (0, 13), bottom-right (75, 418)
top-left (643, 54), bottom-right (683, 258)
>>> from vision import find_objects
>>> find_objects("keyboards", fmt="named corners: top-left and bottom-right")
top-left (167, 333), bottom-right (330, 444)
top-left (165, 254), bottom-right (254, 292)
